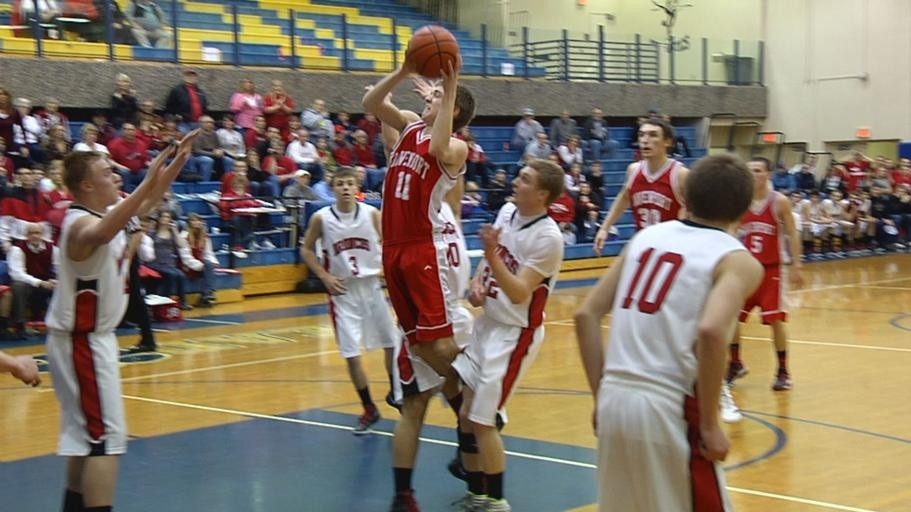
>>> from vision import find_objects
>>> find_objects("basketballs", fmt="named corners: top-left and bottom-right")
top-left (407, 25), bottom-right (458, 79)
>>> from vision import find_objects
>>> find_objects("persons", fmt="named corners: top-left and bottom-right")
top-left (297, 167), bottom-right (403, 437)
top-left (0, 348), bottom-right (43, 388)
top-left (146, 209), bottom-right (194, 314)
top-left (388, 161), bottom-right (476, 512)
top-left (362, 36), bottom-right (508, 484)
top-left (592, 115), bottom-right (747, 427)
top-left (724, 157), bottom-right (808, 394)
top-left (76, 69), bottom-right (220, 217)
top-left (572, 151), bottom-right (766, 512)
top-left (188, 212), bottom-right (219, 309)
top-left (770, 151), bottom-right (911, 263)
top-left (110, 193), bottom-right (157, 352)
top-left (0, 88), bottom-right (75, 343)
top-left (451, 107), bottom-right (691, 246)
top-left (217, 77), bottom-right (387, 263)
top-left (16, 0), bottom-right (174, 48)
top-left (43, 141), bottom-right (192, 512)
top-left (450, 158), bottom-right (566, 511)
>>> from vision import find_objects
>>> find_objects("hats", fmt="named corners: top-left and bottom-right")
top-left (200, 116), bottom-right (214, 123)
top-left (523, 108), bottom-right (535, 115)
top-left (295, 169), bottom-right (311, 178)
top-left (185, 68), bottom-right (198, 75)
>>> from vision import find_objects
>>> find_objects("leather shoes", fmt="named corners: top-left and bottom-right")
top-left (130, 343), bottom-right (157, 352)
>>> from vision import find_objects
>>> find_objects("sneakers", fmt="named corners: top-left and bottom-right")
top-left (353, 407), bottom-right (381, 434)
top-left (249, 242), bottom-right (261, 249)
top-left (860, 251), bottom-right (871, 256)
top-left (808, 254), bottom-right (818, 261)
top-left (386, 394), bottom-right (403, 414)
top-left (830, 253), bottom-right (843, 259)
top-left (884, 242), bottom-right (911, 253)
top-left (203, 295), bottom-right (215, 301)
top-left (472, 498), bottom-right (511, 512)
top-left (846, 252), bottom-right (860, 257)
top-left (873, 249), bottom-right (882, 255)
top-left (878, 248), bottom-right (886, 254)
top-left (261, 241), bottom-right (275, 249)
top-left (390, 489), bottom-right (421, 512)
top-left (800, 254), bottom-right (807, 263)
top-left (724, 362), bottom-right (748, 387)
top-left (448, 457), bottom-right (466, 481)
top-left (816, 254), bottom-right (826, 260)
top-left (851, 250), bottom-right (863, 256)
top-left (179, 303), bottom-right (194, 310)
top-left (825, 253), bottom-right (836, 260)
top-left (838, 252), bottom-right (847, 258)
top-left (448, 492), bottom-right (489, 512)
top-left (718, 384), bottom-right (742, 423)
top-left (862, 249), bottom-right (873, 254)
top-left (773, 369), bottom-right (792, 390)
top-left (198, 301), bottom-right (211, 308)
